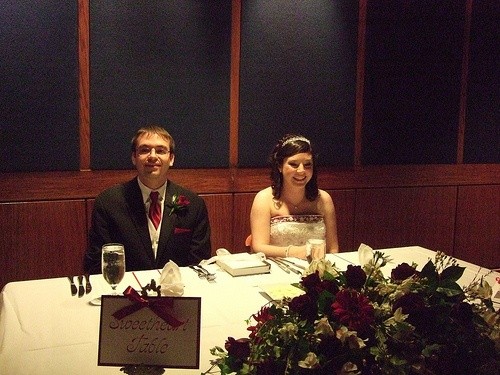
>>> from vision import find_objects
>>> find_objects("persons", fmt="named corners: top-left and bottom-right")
top-left (250, 133), bottom-right (338, 260)
top-left (83, 124), bottom-right (210, 275)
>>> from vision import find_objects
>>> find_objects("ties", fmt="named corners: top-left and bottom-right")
top-left (148, 191), bottom-right (162, 230)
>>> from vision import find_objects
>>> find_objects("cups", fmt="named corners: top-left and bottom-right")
top-left (305, 236), bottom-right (326, 274)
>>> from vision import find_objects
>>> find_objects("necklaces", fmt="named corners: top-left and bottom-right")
top-left (279, 194), bottom-right (309, 210)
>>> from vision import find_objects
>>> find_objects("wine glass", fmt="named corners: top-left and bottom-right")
top-left (101, 243), bottom-right (125, 296)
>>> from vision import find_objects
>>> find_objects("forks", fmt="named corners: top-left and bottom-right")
top-left (188, 264), bottom-right (217, 282)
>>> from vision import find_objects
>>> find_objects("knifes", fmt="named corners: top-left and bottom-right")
top-left (268, 256), bottom-right (307, 275)
top-left (84, 273), bottom-right (92, 294)
top-left (77, 274), bottom-right (85, 298)
top-left (67, 273), bottom-right (77, 296)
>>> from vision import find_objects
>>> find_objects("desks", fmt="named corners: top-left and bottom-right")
top-left (0, 245), bottom-right (500, 375)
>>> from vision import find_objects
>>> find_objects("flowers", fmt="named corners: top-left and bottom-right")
top-left (200, 243), bottom-right (500, 375)
top-left (166, 193), bottom-right (190, 217)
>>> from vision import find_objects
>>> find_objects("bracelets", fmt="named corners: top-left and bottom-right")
top-left (284, 245), bottom-right (291, 258)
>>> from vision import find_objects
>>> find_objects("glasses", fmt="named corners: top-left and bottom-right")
top-left (134, 146), bottom-right (173, 155)
